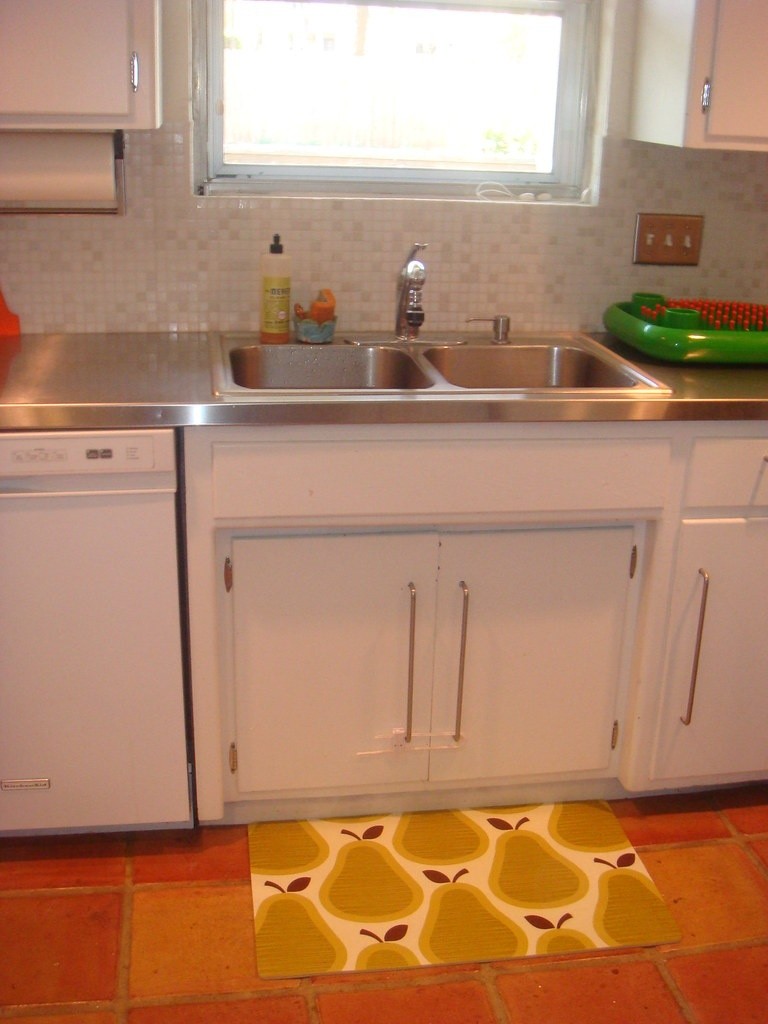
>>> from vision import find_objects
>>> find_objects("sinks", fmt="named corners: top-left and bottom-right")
top-left (422, 343), bottom-right (674, 421)
top-left (211, 340), bottom-right (440, 425)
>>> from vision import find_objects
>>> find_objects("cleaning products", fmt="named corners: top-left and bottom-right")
top-left (258, 232), bottom-right (292, 346)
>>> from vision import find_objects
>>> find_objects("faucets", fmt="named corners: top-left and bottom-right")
top-left (394, 242), bottom-right (431, 343)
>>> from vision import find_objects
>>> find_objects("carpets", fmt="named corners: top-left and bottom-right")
top-left (247, 797), bottom-right (683, 978)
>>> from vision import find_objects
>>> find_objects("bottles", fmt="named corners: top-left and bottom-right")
top-left (260, 234), bottom-right (292, 344)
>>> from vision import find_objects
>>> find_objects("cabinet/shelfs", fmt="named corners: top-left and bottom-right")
top-left (183, 420), bottom-right (683, 827)
top-left (0, 0), bottom-right (162, 133)
top-left (626, 0), bottom-right (767, 152)
top-left (0, 427), bottom-right (194, 839)
top-left (616, 419), bottom-right (767, 797)
